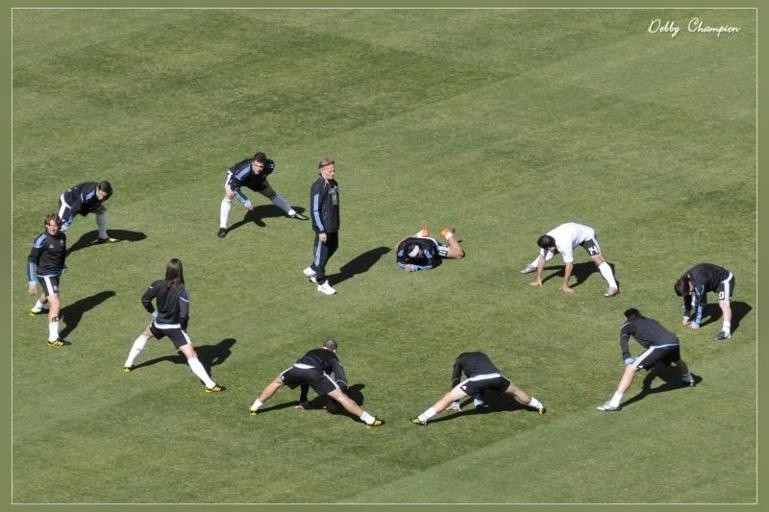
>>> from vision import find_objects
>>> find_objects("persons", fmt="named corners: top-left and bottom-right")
top-left (674, 262), bottom-right (737, 342)
top-left (216, 153), bottom-right (308, 239)
top-left (249, 340), bottom-right (386, 428)
top-left (408, 350), bottom-right (546, 426)
top-left (27, 213), bottom-right (71, 347)
top-left (595, 309), bottom-right (694, 411)
top-left (395, 224), bottom-right (465, 272)
top-left (298, 158), bottom-right (341, 296)
top-left (51, 181), bottom-right (119, 246)
top-left (519, 222), bottom-right (619, 298)
top-left (123, 259), bottom-right (226, 393)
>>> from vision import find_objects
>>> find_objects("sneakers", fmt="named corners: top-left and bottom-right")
top-left (30, 308), bottom-right (50, 313)
top-left (367, 417), bottom-right (383, 426)
top-left (303, 267), bottom-right (318, 284)
top-left (604, 287), bottom-right (618, 296)
top-left (206, 384), bottom-right (224, 393)
top-left (410, 417), bottom-right (427, 425)
top-left (714, 331), bottom-right (732, 340)
top-left (100, 235), bottom-right (118, 243)
top-left (219, 228), bottom-right (226, 237)
top-left (520, 265), bottom-right (536, 274)
top-left (317, 279), bottom-right (336, 295)
top-left (124, 366), bottom-right (130, 372)
top-left (679, 374), bottom-right (694, 383)
top-left (596, 401), bottom-right (621, 411)
top-left (438, 226), bottom-right (455, 236)
top-left (289, 211), bottom-right (309, 221)
top-left (538, 404), bottom-right (543, 414)
top-left (48, 338), bottom-right (71, 347)
top-left (423, 225), bottom-right (429, 237)
top-left (250, 407), bottom-right (257, 415)
